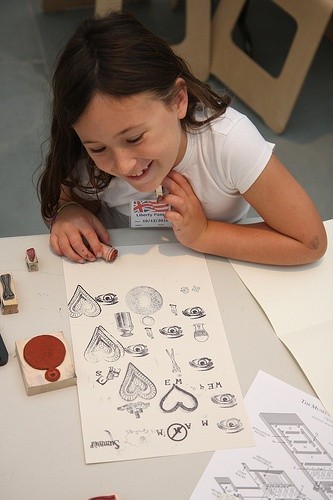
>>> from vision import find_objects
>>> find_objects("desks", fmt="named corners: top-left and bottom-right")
top-left (0, 221), bottom-right (333, 499)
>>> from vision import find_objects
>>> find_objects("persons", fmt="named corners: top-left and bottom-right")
top-left (34, 9), bottom-right (330, 265)
